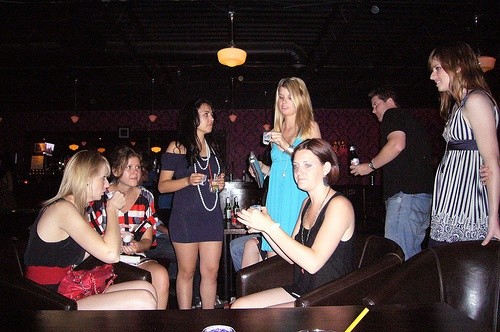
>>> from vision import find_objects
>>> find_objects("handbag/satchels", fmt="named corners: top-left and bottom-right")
top-left (57, 263), bottom-right (116, 302)
top-left (134, 233), bottom-right (175, 260)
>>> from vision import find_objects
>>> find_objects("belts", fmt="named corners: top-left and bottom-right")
top-left (25, 265), bottom-right (75, 284)
top-left (447, 140), bottom-right (478, 150)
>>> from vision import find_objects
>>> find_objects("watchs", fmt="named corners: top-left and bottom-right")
top-left (368, 161), bottom-right (377, 171)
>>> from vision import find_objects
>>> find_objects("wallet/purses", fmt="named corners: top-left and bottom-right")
top-left (248, 155), bottom-right (264, 188)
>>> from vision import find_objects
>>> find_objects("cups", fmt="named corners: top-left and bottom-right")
top-left (210, 173), bottom-right (218, 192)
top-left (250, 205), bottom-right (261, 212)
top-left (202, 325), bottom-right (236, 332)
top-left (263, 132), bottom-right (271, 144)
top-left (106, 191), bottom-right (114, 199)
top-left (298, 329), bottom-right (336, 332)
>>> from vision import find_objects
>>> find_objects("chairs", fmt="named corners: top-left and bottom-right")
top-left (235, 234), bottom-right (404, 307)
top-left (361, 240), bottom-right (500, 332)
top-left (0, 238), bottom-right (152, 310)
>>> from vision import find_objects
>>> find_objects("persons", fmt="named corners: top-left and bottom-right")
top-left (23, 150), bottom-right (157, 310)
top-left (230, 76), bottom-right (321, 272)
top-left (83, 146), bottom-right (170, 310)
top-left (230, 137), bottom-right (357, 309)
top-left (158, 100), bottom-right (225, 310)
top-left (349, 86), bottom-right (435, 261)
top-left (428, 41), bottom-right (500, 246)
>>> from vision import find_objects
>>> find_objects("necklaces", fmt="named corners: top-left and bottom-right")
top-left (194, 138), bottom-right (220, 212)
top-left (301, 187), bottom-right (331, 274)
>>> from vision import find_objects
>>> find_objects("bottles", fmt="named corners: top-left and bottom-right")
top-left (233, 196), bottom-right (239, 218)
top-left (349, 142), bottom-right (361, 177)
top-left (224, 197), bottom-right (231, 222)
top-left (242, 170), bottom-right (246, 182)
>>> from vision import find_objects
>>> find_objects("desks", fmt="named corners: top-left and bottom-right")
top-left (223, 218), bottom-right (247, 300)
top-left (0, 302), bottom-right (495, 332)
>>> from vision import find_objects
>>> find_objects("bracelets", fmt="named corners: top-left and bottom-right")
top-left (284, 145), bottom-right (295, 156)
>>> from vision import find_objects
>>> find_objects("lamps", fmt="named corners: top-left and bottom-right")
top-left (70, 85), bottom-right (79, 123)
top-left (148, 78), bottom-right (157, 122)
top-left (228, 76), bottom-right (237, 122)
top-left (263, 97), bottom-right (270, 131)
top-left (217, 9), bottom-right (247, 67)
